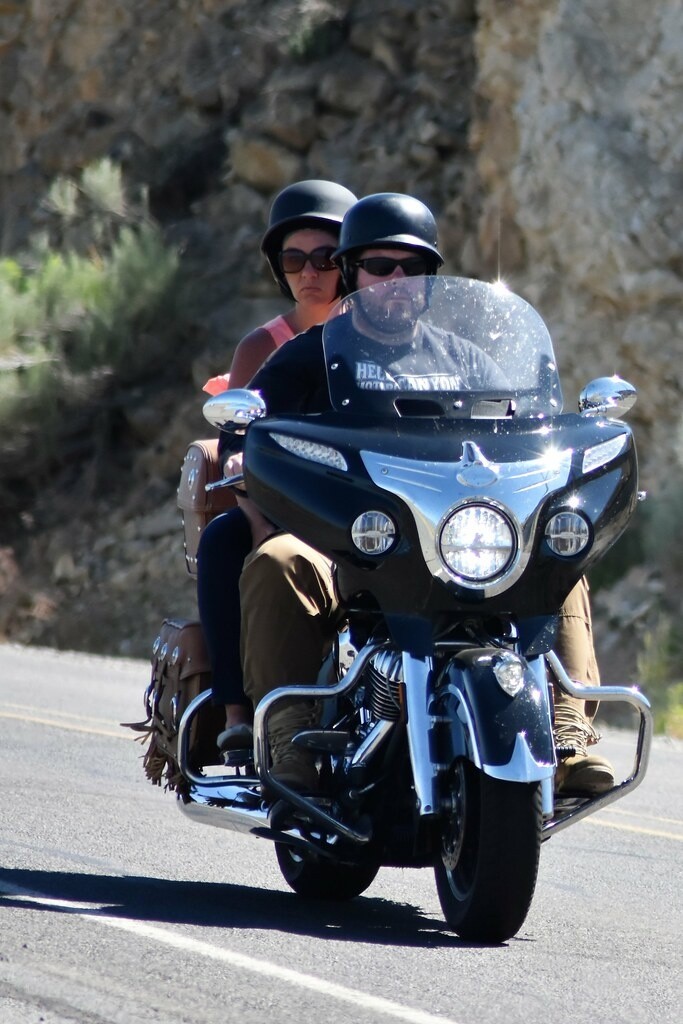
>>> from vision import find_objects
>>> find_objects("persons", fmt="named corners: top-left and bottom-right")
top-left (215, 191), bottom-right (616, 799)
top-left (194, 177), bottom-right (358, 751)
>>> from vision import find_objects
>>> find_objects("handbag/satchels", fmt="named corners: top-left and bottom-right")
top-left (120, 617), bottom-right (227, 797)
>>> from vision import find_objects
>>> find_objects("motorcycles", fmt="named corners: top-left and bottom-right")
top-left (174, 274), bottom-right (651, 945)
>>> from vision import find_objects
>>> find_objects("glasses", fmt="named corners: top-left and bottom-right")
top-left (278, 246), bottom-right (340, 273)
top-left (351, 256), bottom-right (430, 276)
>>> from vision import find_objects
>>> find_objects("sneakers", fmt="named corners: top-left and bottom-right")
top-left (552, 693), bottom-right (614, 811)
top-left (260, 700), bottom-right (322, 801)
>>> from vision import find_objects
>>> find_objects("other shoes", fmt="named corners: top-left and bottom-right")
top-left (217, 723), bottom-right (253, 767)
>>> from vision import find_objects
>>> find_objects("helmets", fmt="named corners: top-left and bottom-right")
top-left (329, 192), bottom-right (444, 288)
top-left (260, 180), bottom-right (359, 298)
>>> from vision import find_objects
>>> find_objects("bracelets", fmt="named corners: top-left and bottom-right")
top-left (223, 475), bottom-right (250, 498)
